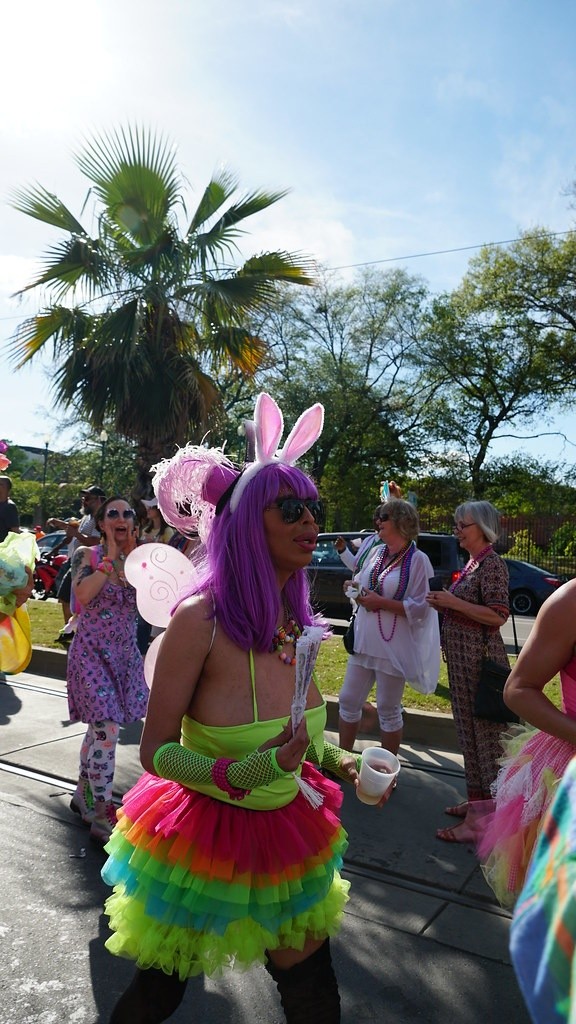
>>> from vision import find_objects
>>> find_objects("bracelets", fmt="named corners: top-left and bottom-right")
top-left (212, 757), bottom-right (252, 800)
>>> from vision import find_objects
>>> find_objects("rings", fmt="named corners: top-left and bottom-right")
top-left (434, 594), bottom-right (437, 599)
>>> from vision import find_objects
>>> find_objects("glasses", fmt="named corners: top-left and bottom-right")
top-left (101, 509), bottom-right (136, 520)
top-left (262, 499), bottom-right (325, 524)
top-left (457, 519), bottom-right (477, 531)
top-left (82, 496), bottom-right (98, 502)
top-left (378, 514), bottom-right (396, 522)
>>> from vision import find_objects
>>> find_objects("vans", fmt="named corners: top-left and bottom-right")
top-left (305, 530), bottom-right (466, 619)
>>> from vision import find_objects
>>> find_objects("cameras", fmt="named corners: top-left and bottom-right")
top-left (429, 577), bottom-right (443, 593)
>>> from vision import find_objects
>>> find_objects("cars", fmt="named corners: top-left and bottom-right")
top-left (32, 531), bottom-right (69, 559)
top-left (502, 558), bottom-right (566, 615)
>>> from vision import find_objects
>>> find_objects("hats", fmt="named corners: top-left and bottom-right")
top-left (80, 486), bottom-right (106, 504)
top-left (141, 497), bottom-right (159, 508)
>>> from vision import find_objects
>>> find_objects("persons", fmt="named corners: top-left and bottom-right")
top-left (332, 480), bottom-right (404, 612)
top-left (0, 477), bottom-right (33, 686)
top-left (108, 462), bottom-right (400, 1024)
top-left (33, 485), bottom-right (207, 651)
top-left (466, 575), bottom-right (576, 1024)
top-left (337, 500), bottom-right (441, 755)
top-left (426, 500), bottom-right (524, 844)
top-left (68, 497), bottom-right (149, 844)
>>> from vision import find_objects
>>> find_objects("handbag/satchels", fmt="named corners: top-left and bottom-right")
top-left (343, 614), bottom-right (357, 655)
top-left (472, 659), bottom-right (521, 722)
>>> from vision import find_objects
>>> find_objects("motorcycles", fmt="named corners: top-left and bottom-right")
top-left (27, 552), bottom-right (71, 601)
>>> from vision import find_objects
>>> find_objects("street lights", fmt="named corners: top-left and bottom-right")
top-left (99, 429), bottom-right (108, 488)
top-left (43, 433), bottom-right (52, 501)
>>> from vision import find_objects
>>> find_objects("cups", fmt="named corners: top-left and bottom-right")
top-left (355, 747), bottom-right (401, 806)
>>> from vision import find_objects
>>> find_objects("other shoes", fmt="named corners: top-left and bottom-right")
top-left (55, 630), bottom-right (75, 642)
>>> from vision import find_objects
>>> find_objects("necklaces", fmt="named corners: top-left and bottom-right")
top-left (370, 540), bottom-right (418, 641)
top-left (270, 589), bottom-right (304, 666)
top-left (441, 544), bottom-right (494, 662)
top-left (102, 541), bottom-right (132, 589)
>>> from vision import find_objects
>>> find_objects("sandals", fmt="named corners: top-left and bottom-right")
top-left (444, 801), bottom-right (486, 816)
top-left (436, 820), bottom-right (480, 842)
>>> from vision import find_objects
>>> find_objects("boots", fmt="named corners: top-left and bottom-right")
top-left (70, 777), bottom-right (95, 824)
top-left (108, 953), bottom-right (196, 1024)
top-left (89, 800), bottom-right (118, 844)
top-left (264, 933), bottom-right (342, 1024)
